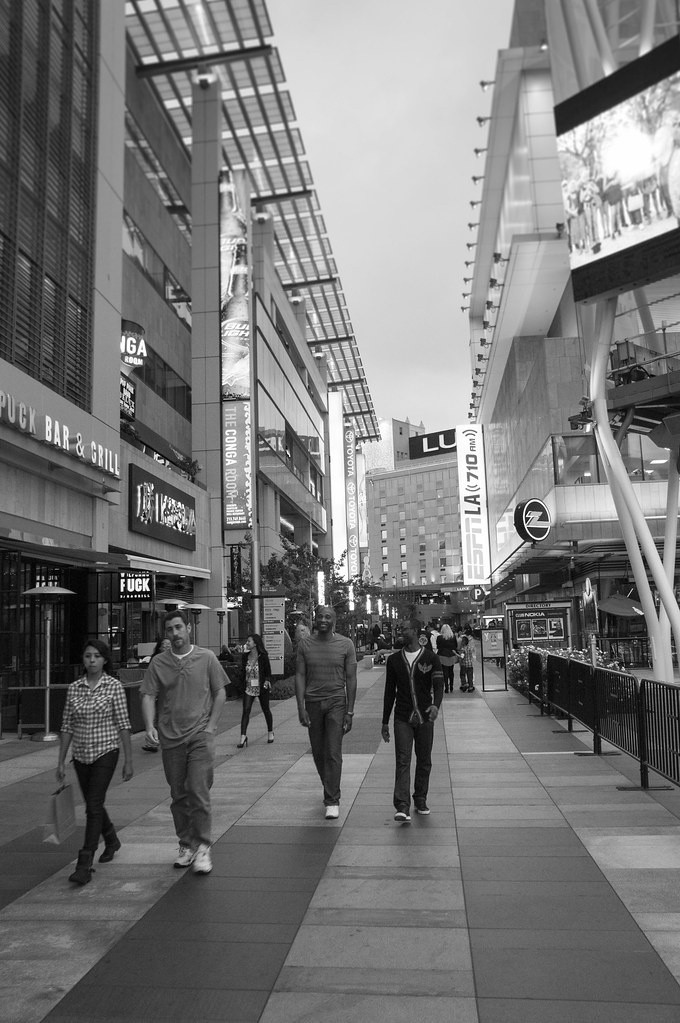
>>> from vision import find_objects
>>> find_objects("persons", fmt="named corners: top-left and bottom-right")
top-left (218, 644), bottom-right (234, 662)
top-left (295, 605), bottom-right (357, 819)
top-left (237, 634), bottom-right (274, 748)
top-left (372, 622), bottom-right (404, 663)
top-left (425, 619), bottom-right (506, 694)
top-left (139, 610), bottom-right (231, 874)
top-left (553, 622), bottom-right (562, 636)
top-left (562, 109), bottom-right (680, 256)
top-left (56, 640), bottom-right (134, 883)
top-left (142, 639), bottom-right (172, 752)
top-left (381, 619), bottom-right (444, 821)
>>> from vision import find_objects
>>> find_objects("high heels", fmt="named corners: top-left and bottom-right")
top-left (267, 732), bottom-right (274, 743)
top-left (237, 736), bottom-right (248, 748)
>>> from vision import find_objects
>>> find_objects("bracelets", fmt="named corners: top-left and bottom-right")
top-left (345, 712), bottom-right (354, 715)
top-left (208, 726), bottom-right (217, 731)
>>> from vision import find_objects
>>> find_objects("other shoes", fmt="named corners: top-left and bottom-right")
top-left (450, 687), bottom-right (453, 692)
top-left (445, 689), bottom-right (449, 693)
top-left (468, 687), bottom-right (475, 693)
top-left (141, 744), bottom-right (157, 752)
top-left (460, 685), bottom-right (467, 692)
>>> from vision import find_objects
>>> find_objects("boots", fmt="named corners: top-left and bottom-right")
top-left (98, 823), bottom-right (121, 863)
top-left (69, 849), bottom-right (97, 884)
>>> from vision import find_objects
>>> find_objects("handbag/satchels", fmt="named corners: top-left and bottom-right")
top-left (42, 778), bottom-right (77, 846)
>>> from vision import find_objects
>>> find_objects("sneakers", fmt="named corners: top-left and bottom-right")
top-left (414, 800), bottom-right (429, 814)
top-left (325, 804), bottom-right (340, 818)
top-left (393, 810), bottom-right (411, 822)
top-left (173, 847), bottom-right (195, 870)
top-left (193, 847), bottom-right (213, 873)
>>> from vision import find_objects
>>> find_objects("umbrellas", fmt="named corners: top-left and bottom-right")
top-left (597, 593), bottom-right (644, 637)
